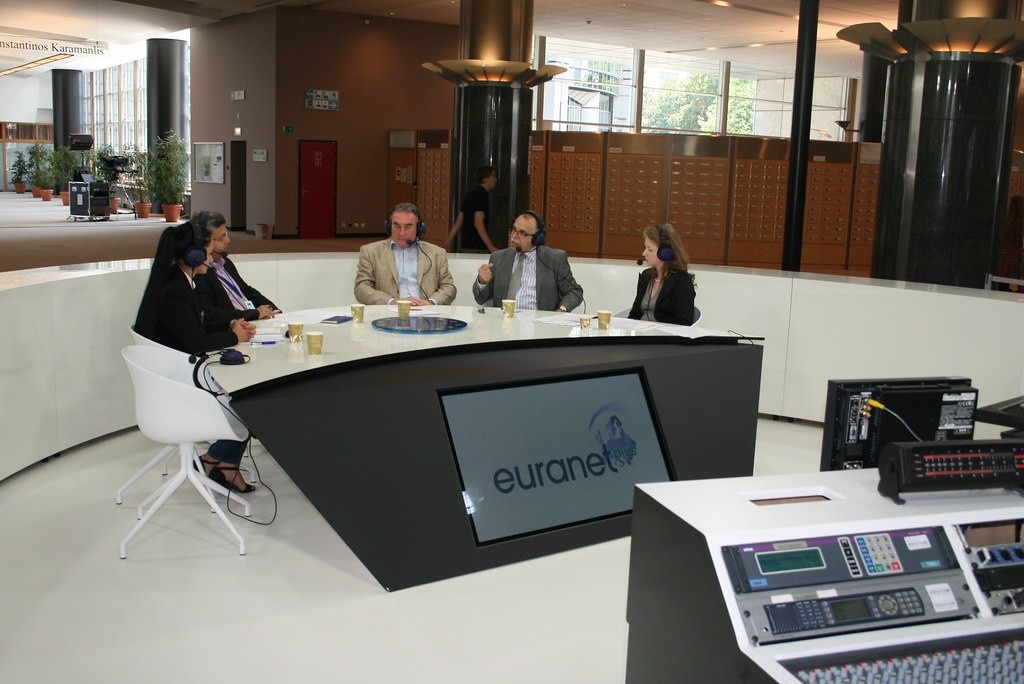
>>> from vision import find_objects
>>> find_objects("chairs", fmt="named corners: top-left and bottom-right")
top-left (484, 295), bottom-right (586, 315)
top-left (614, 305), bottom-right (702, 327)
top-left (116, 324), bottom-right (256, 560)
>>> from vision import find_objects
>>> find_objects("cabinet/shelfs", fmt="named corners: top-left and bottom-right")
top-left (415, 128), bottom-right (882, 277)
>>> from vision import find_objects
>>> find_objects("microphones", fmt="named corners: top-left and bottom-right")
top-left (637, 259), bottom-right (646, 265)
top-left (188, 348), bottom-right (228, 364)
top-left (407, 236), bottom-right (421, 247)
top-left (515, 246), bottom-right (540, 253)
top-left (202, 263), bottom-right (216, 273)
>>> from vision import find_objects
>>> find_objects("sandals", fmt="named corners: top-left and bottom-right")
top-left (193, 456), bottom-right (220, 477)
top-left (206, 465), bottom-right (256, 492)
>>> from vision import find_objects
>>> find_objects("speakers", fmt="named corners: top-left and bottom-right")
top-left (69, 133), bottom-right (93, 150)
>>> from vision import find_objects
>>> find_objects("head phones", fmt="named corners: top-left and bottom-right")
top-left (384, 208), bottom-right (427, 237)
top-left (509, 210), bottom-right (547, 246)
top-left (192, 348), bottom-right (245, 398)
top-left (653, 223), bottom-right (674, 262)
top-left (183, 220), bottom-right (207, 267)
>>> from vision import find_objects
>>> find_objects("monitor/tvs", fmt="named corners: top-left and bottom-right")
top-left (820, 375), bottom-right (980, 474)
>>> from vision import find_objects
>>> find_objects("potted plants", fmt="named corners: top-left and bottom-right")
top-left (7, 127), bottom-right (191, 222)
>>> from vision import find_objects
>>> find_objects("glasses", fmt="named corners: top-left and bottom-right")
top-left (214, 230), bottom-right (229, 242)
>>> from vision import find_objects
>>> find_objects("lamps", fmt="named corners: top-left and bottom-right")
top-left (835, 121), bottom-right (859, 132)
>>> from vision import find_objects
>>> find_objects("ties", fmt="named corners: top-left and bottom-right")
top-left (505, 252), bottom-right (525, 300)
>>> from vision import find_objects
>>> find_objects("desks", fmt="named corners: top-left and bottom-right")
top-left (203, 305), bottom-right (765, 592)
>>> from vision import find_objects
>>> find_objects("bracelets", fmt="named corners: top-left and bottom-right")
top-left (490, 248), bottom-right (496, 252)
top-left (560, 306), bottom-right (566, 311)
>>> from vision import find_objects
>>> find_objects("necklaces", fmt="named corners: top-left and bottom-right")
top-left (655, 279), bottom-right (660, 281)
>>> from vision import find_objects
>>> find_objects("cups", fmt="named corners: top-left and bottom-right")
top-left (580, 316), bottom-right (591, 329)
top-left (288, 322), bottom-right (304, 343)
top-left (502, 300), bottom-right (515, 320)
top-left (307, 331), bottom-right (324, 354)
top-left (397, 300), bottom-right (411, 320)
top-left (598, 311), bottom-right (612, 330)
top-left (351, 303), bottom-right (364, 324)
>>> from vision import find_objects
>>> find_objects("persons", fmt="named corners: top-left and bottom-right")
top-left (134, 221), bottom-right (257, 493)
top-left (443, 165), bottom-right (497, 254)
top-left (473, 211), bottom-right (584, 313)
top-left (628, 223), bottom-right (696, 325)
top-left (190, 211), bottom-right (283, 322)
top-left (354, 203), bottom-right (457, 305)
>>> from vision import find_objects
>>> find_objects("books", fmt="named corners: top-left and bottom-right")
top-left (322, 316), bottom-right (352, 324)
